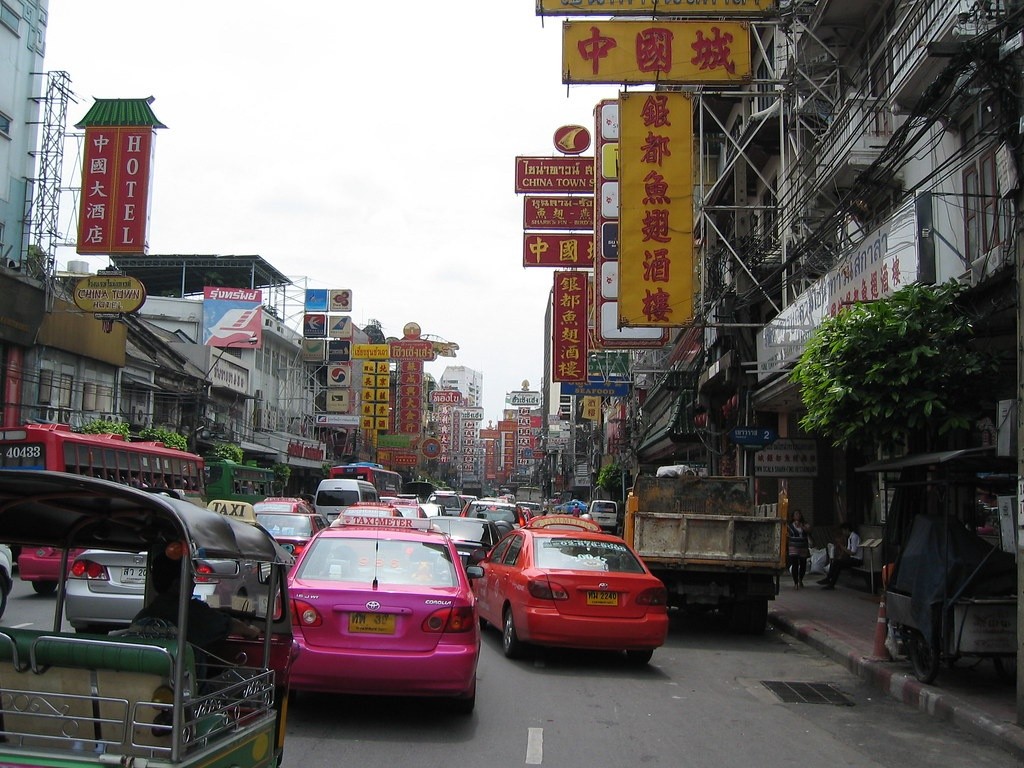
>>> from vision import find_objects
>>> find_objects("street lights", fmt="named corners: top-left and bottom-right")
top-left (192, 337), bottom-right (258, 455)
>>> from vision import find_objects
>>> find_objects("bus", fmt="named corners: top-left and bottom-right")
top-left (329, 462), bottom-right (403, 496)
top-left (404, 482), bottom-right (442, 502)
top-left (0, 424), bottom-right (206, 500)
top-left (186, 457), bottom-right (279, 505)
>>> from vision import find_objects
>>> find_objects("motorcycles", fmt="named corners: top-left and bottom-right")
top-left (0, 468), bottom-right (299, 768)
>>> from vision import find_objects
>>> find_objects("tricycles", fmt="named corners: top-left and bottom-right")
top-left (854, 447), bottom-right (1019, 683)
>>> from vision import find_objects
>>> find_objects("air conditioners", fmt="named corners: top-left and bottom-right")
top-left (101, 414), bottom-right (116, 425)
top-left (117, 416), bottom-right (124, 426)
top-left (129, 404), bottom-right (147, 427)
top-left (59, 407), bottom-right (73, 424)
top-left (268, 411), bottom-right (277, 431)
top-left (40, 405), bottom-right (59, 423)
top-left (256, 408), bottom-right (268, 430)
top-left (5, 257), bottom-right (20, 269)
top-left (256, 389), bottom-right (265, 400)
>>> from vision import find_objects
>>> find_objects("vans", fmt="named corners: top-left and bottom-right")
top-left (313, 478), bottom-right (380, 523)
top-left (589, 499), bottom-right (618, 534)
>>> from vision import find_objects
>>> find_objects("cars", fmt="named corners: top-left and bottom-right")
top-left (277, 507), bottom-right (485, 715)
top-left (0, 544), bottom-right (13, 618)
top-left (552, 499), bottom-right (589, 515)
top-left (469, 526), bottom-right (670, 667)
top-left (65, 499), bottom-right (294, 635)
top-left (18, 545), bottom-right (89, 595)
top-left (380, 490), bottom-right (543, 533)
top-left (417, 516), bottom-right (503, 566)
top-left (253, 497), bottom-right (331, 556)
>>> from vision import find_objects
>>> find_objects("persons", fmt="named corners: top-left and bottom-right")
top-left (73, 467), bottom-right (201, 491)
top-left (596, 504), bottom-right (602, 511)
top-left (543, 496), bottom-right (561, 515)
top-left (787, 509), bottom-right (811, 590)
top-left (572, 503), bottom-right (581, 518)
top-left (816, 522), bottom-right (864, 590)
top-left (130, 550), bottom-right (263, 712)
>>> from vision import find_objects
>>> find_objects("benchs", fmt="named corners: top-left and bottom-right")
top-left (0, 624), bottom-right (237, 758)
top-left (830, 524), bottom-right (884, 594)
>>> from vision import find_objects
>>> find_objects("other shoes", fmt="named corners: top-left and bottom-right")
top-left (794, 582), bottom-right (798, 589)
top-left (815, 579), bottom-right (830, 591)
top-left (798, 579), bottom-right (803, 587)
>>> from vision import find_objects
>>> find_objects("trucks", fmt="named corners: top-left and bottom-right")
top-left (622, 471), bottom-right (791, 634)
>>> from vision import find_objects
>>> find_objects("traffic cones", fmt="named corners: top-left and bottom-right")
top-left (865, 593), bottom-right (901, 664)
